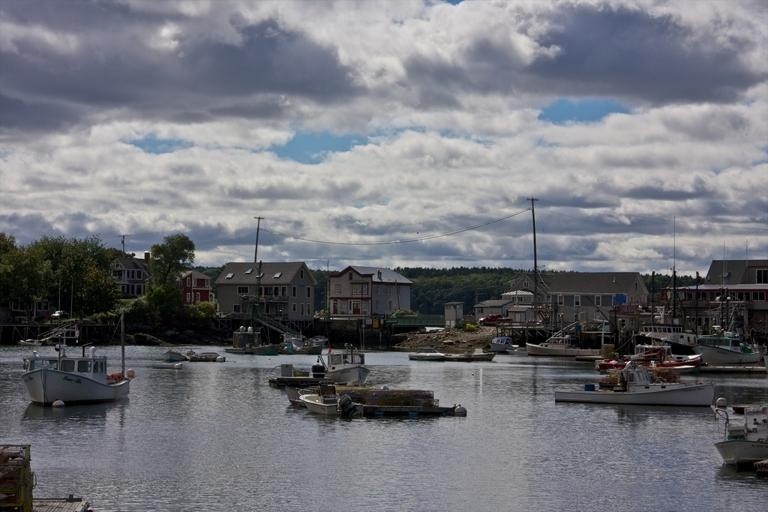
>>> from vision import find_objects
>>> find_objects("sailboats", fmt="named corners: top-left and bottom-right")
top-left (223, 257), bottom-right (282, 357)
top-left (605, 215), bottom-right (765, 365)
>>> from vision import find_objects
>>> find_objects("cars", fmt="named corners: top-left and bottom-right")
top-left (51, 309), bottom-right (68, 319)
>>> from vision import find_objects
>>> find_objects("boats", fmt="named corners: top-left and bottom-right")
top-left (525, 315), bottom-right (600, 357)
top-left (707, 397), bottom-right (767, 468)
top-left (191, 350), bottom-right (228, 363)
top-left (17, 318), bottom-right (138, 405)
top-left (479, 335), bottom-right (516, 355)
top-left (406, 350), bottom-right (496, 363)
top-left (276, 331), bottom-right (324, 355)
top-left (593, 342), bottom-right (710, 374)
top-left (553, 354), bottom-right (715, 408)
top-left (160, 350), bottom-right (187, 362)
top-left (266, 341), bottom-right (470, 420)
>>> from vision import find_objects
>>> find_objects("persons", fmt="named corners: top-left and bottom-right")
top-left (753, 418), bottom-right (760, 425)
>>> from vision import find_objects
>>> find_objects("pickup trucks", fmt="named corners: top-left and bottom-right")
top-left (475, 312), bottom-right (514, 328)
